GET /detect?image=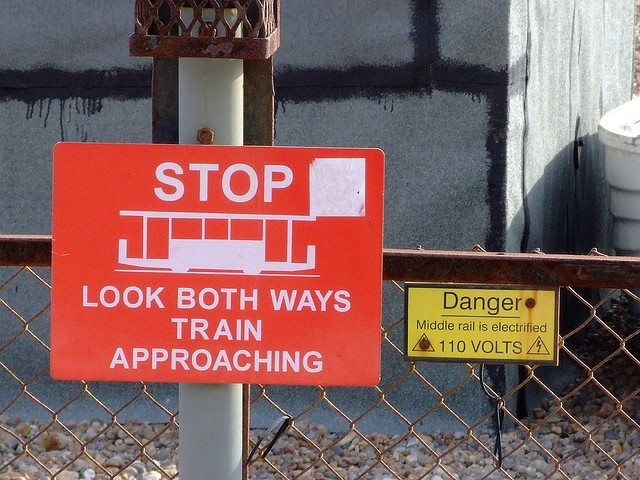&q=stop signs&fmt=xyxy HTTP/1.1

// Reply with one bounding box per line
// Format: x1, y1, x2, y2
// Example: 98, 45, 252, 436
51, 142, 384, 386
